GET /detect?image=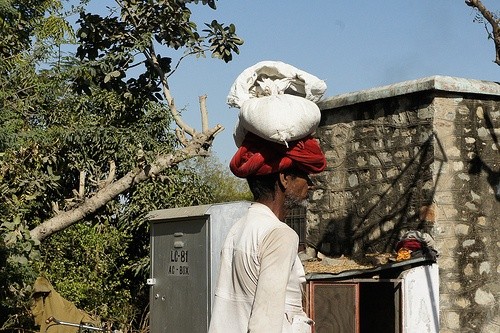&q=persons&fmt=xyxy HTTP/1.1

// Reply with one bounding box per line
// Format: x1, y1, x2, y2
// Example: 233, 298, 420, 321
205, 111, 328, 333
